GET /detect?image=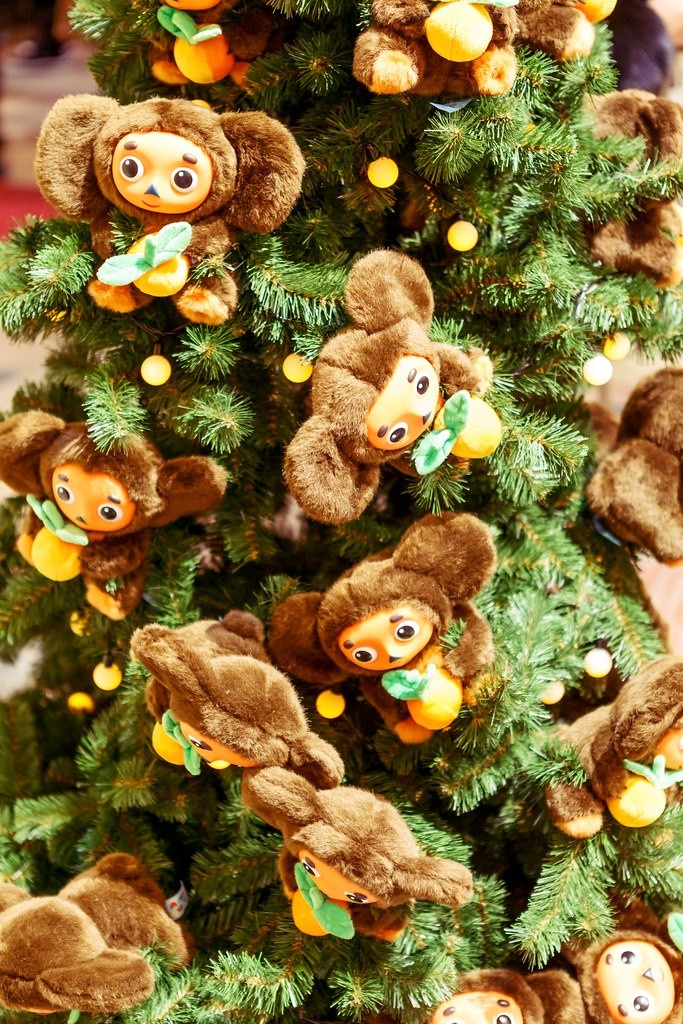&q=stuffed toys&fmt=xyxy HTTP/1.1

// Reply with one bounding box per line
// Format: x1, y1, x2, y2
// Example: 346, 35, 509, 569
425, 894, 682, 1024
587, 367, 682, 563
579, 89, 683, 286
35, 95, 304, 325
131, 610, 345, 790
545, 656, 682, 839
1, 853, 194, 1015
270, 511, 496, 743
358, 0, 622, 98
141, 2, 286, 90
284, 250, 504, 527
242, 767, 474, 943
1, 410, 226, 620
